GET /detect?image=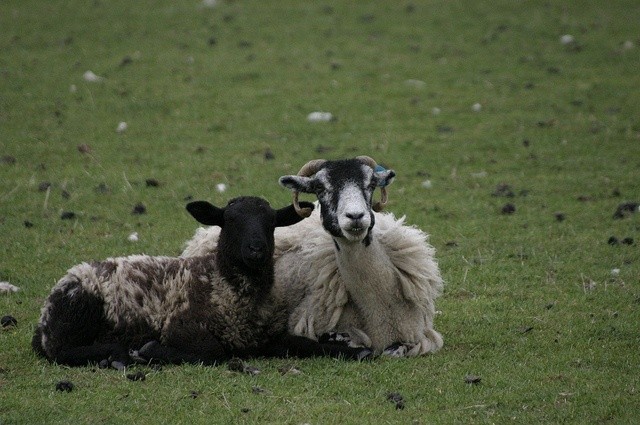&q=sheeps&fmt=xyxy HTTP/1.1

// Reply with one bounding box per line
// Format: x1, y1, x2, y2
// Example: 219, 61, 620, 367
174, 155, 442, 360
32, 196, 316, 371
